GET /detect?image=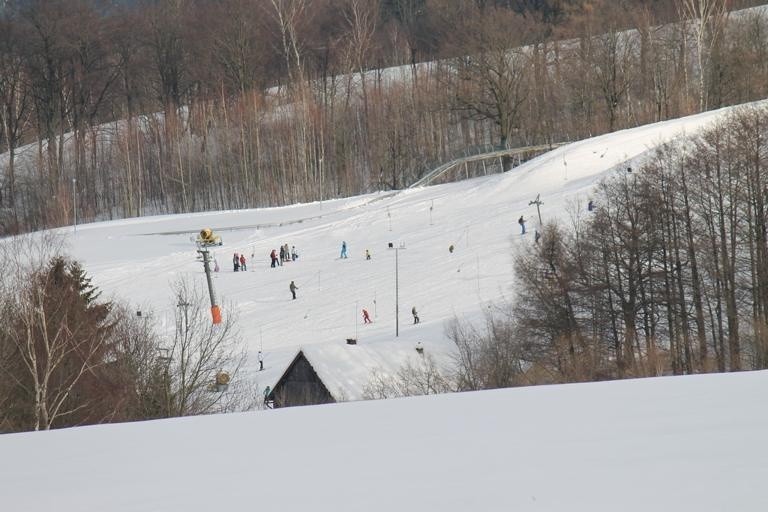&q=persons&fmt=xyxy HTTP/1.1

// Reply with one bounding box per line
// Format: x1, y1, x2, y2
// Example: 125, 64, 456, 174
365, 249, 371, 260
361, 309, 373, 325
232, 253, 247, 272
519, 213, 527, 234
340, 241, 347, 259
290, 281, 299, 300
588, 200, 596, 216
412, 306, 420, 324
257, 350, 264, 372
270, 244, 298, 268
